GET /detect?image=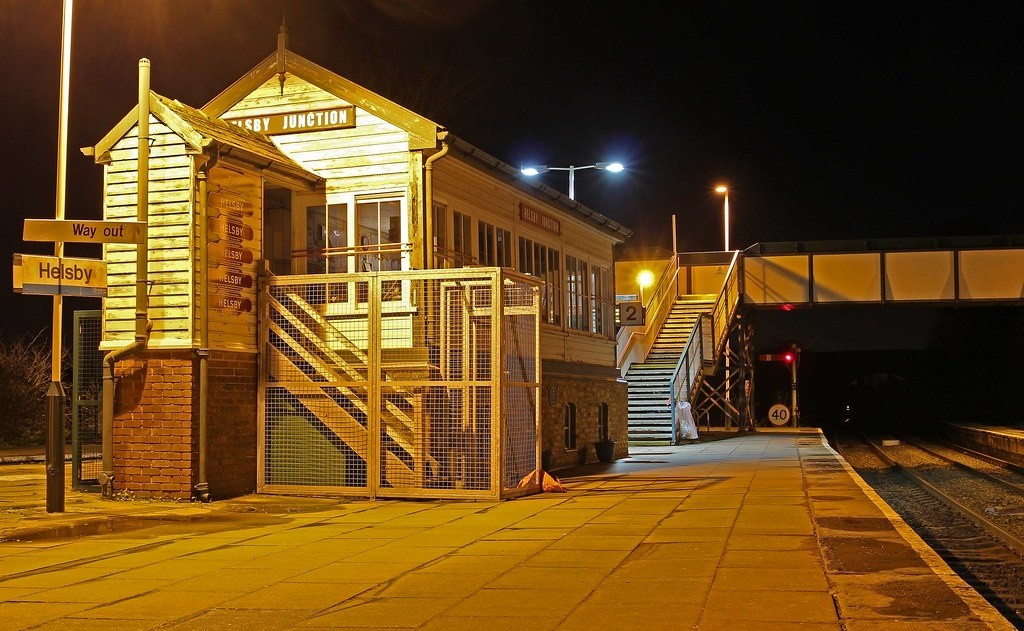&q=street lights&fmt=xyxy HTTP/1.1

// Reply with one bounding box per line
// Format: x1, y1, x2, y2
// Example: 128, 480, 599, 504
518, 160, 623, 200
635, 271, 653, 307
716, 187, 730, 253
